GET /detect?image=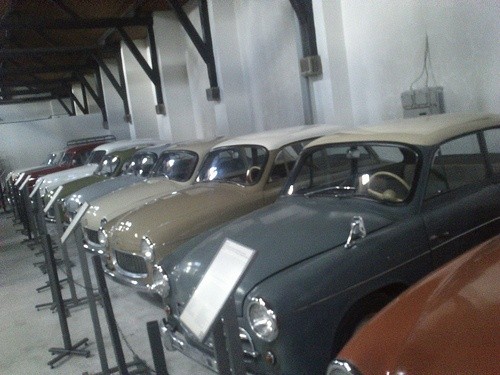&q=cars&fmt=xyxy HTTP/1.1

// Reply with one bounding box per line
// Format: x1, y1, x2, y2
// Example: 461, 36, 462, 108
5, 112, 500, 375
